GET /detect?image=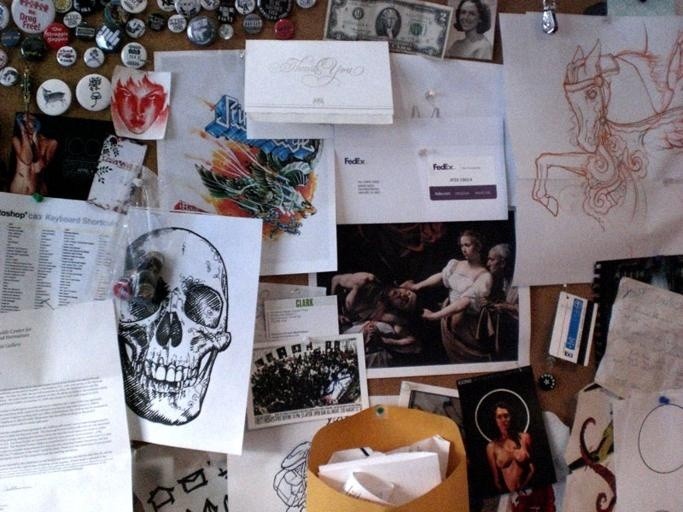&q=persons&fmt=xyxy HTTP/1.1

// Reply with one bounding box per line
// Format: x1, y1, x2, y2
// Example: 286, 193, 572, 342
446, 0, 493, 61
332, 229, 518, 363
486, 399, 537, 511
381, 9, 397, 37
252, 345, 359, 415
10, 113, 59, 195
110, 65, 171, 135
